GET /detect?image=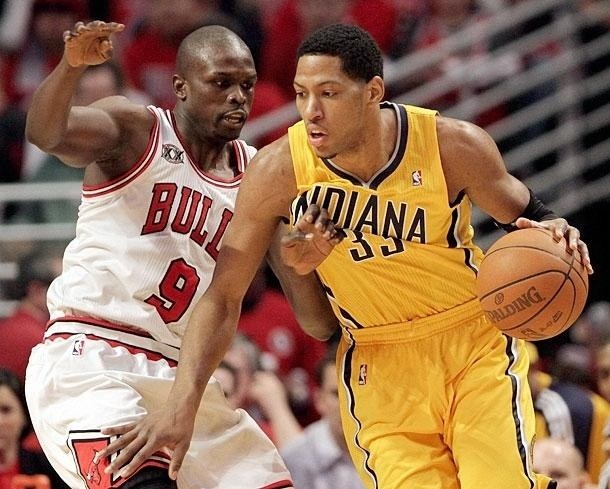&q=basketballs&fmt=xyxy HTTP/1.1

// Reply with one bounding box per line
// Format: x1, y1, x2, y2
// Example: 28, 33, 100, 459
477, 228, 590, 343
